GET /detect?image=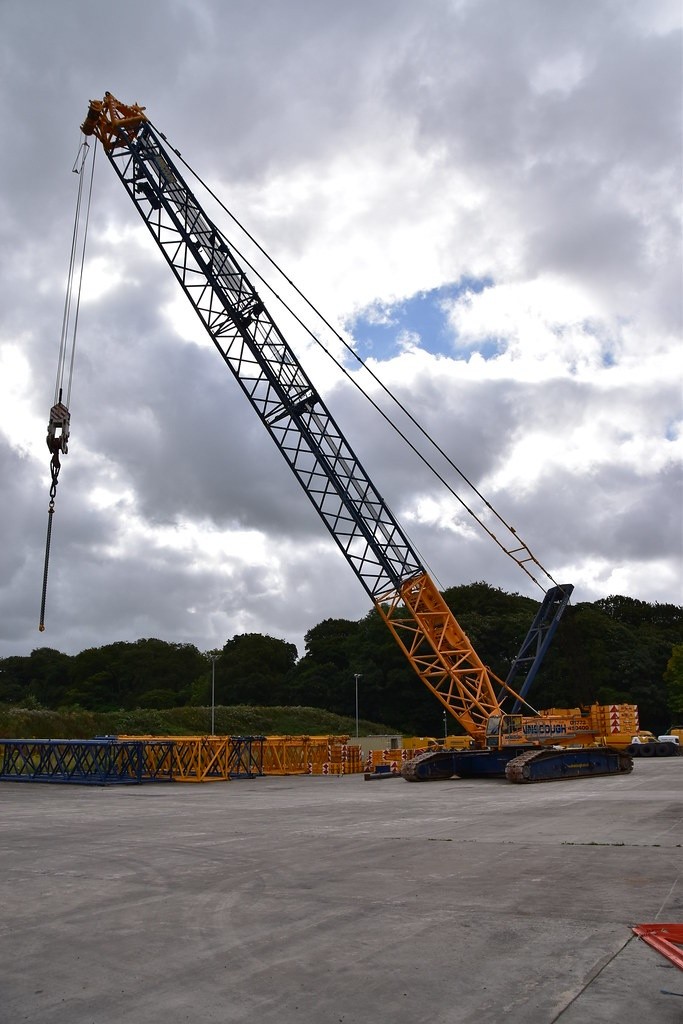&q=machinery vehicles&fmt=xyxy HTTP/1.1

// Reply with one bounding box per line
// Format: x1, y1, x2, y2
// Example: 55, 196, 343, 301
35, 89, 634, 785
